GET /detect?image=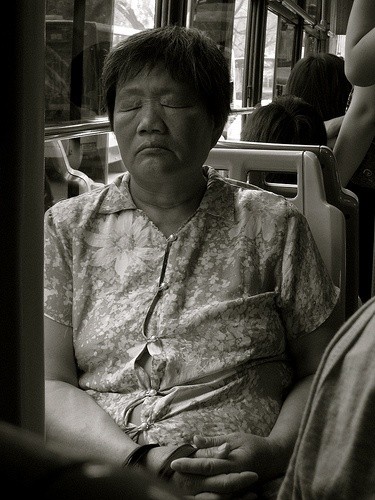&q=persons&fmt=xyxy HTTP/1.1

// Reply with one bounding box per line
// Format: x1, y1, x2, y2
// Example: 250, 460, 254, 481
44, 26, 342, 500
333, 86, 375, 188
284, 53, 353, 122
241, 96, 363, 313
344, 0, 375, 86
